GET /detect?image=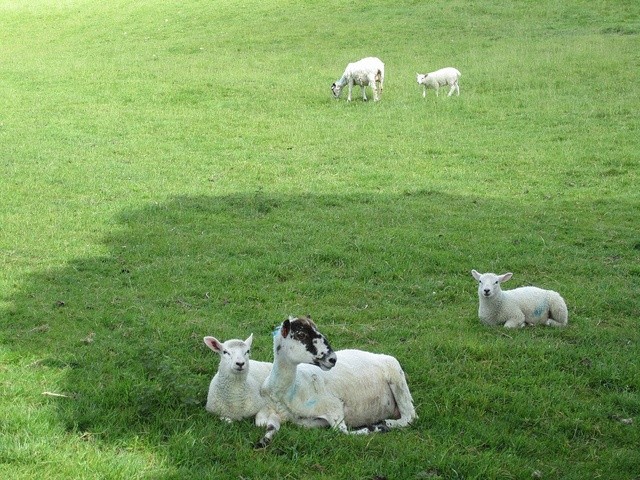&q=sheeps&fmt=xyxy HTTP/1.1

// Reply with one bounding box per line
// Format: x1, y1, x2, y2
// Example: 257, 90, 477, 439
255, 313, 419, 450
204, 333, 273, 425
331, 56, 462, 102
471, 268, 570, 328
